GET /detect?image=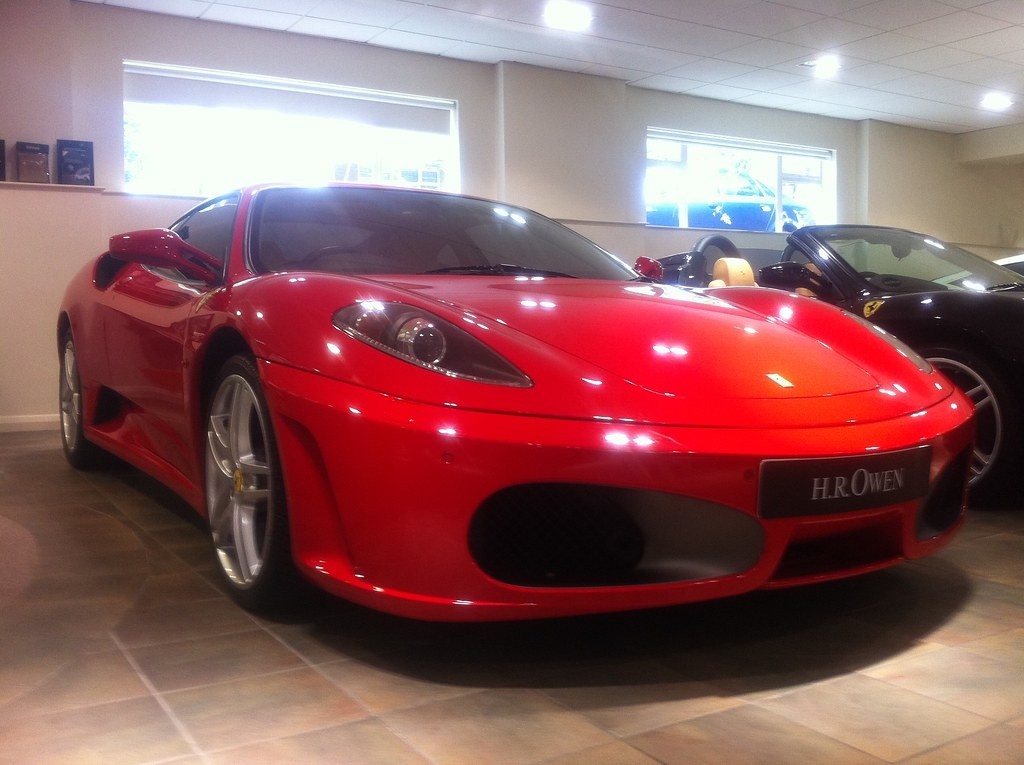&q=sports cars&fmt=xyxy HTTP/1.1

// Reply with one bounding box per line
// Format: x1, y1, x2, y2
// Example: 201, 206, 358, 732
636, 218, 1024, 523
51, 175, 981, 621
